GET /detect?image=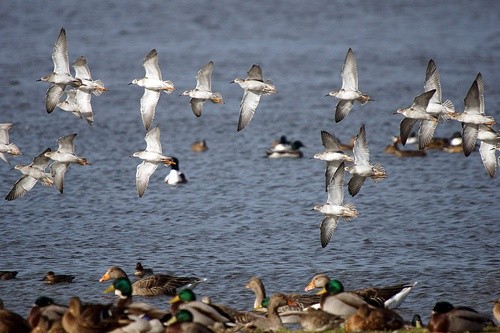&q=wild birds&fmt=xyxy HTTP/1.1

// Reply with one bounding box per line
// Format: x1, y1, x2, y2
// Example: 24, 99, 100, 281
37, 27, 111, 126
309, 123, 387, 198
264, 135, 308, 160
192, 140, 209, 153
0, 261, 500, 333
165, 156, 188, 186
386, 58, 500, 179
128, 125, 178, 199
128, 48, 177, 131
180, 61, 226, 117
0, 122, 23, 165
309, 162, 361, 249
4, 131, 87, 202
228, 63, 279, 132
324, 47, 375, 124
